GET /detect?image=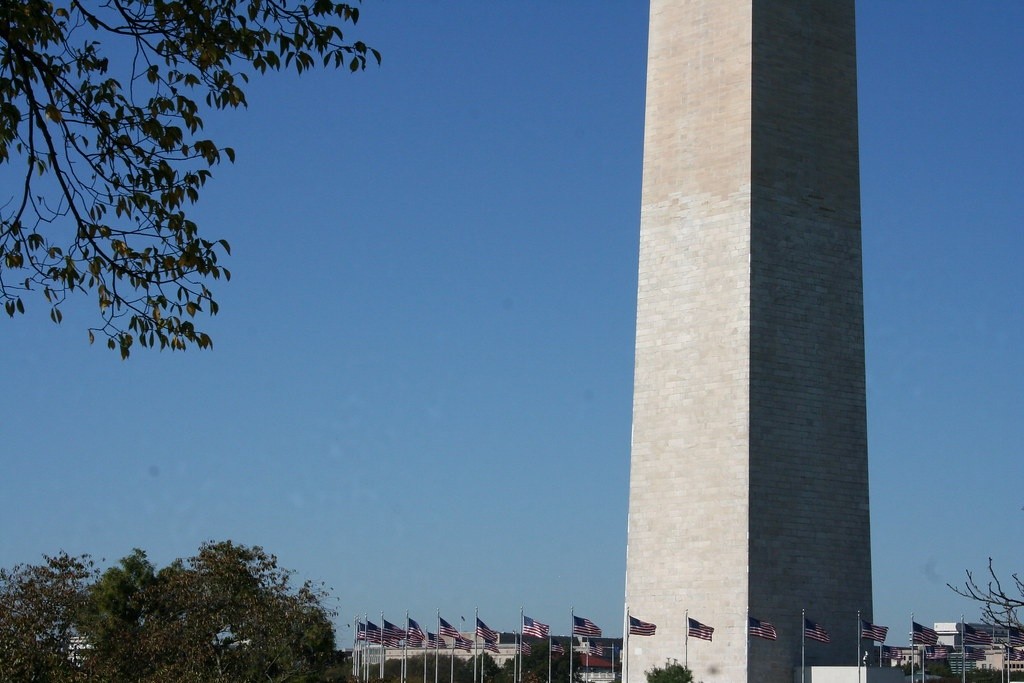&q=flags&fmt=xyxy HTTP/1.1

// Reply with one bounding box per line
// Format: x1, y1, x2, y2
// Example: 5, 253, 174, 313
355, 620, 406, 648
517, 639, 531, 656
688, 617, 714, 642
1003, 628, 1024, 661
881, 644, 903, 660
861, 620, 889, 644
588, 638, 604, 656
477, 618, 500, 653
552, 638, 564, 655
573, 615, 602, 637
629, 615, 657, 636
427, 632, 447, 649
439, 617, 474, 651
407, 618, 425, 647
804, 618, 831, 643
522, 615, 549, 640
964, 623, 994, 661
913, 621, 948, 659
749, 617, 776, 641
